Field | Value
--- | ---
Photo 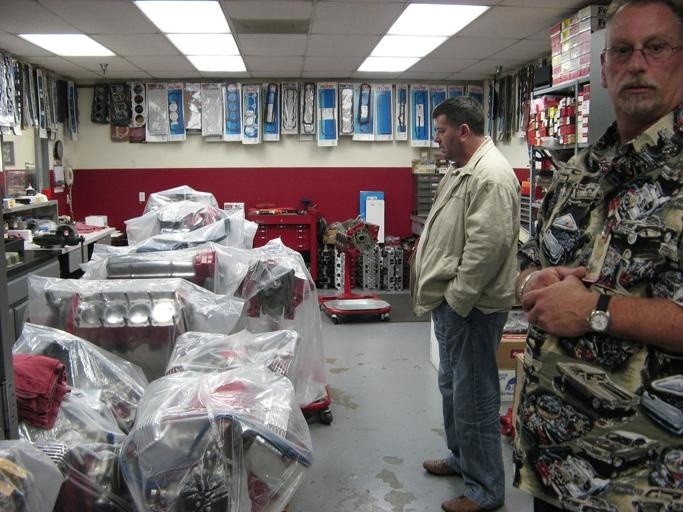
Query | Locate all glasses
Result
[601,40,682,63]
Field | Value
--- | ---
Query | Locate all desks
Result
[0,224,118,438]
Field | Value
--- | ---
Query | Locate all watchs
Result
[585,292,615,335]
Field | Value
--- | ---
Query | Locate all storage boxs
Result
[428,311,530,402]
[551,5,608,85]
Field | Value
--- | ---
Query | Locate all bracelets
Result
[516,268,539,300]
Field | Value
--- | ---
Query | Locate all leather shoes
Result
[442,495,484,511]
[424,457,461,476]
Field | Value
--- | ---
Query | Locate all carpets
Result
[325,292,432,324]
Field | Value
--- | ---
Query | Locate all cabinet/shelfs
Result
[519,193,532,232]
[244,209,318,289]
[525,75,593,252]
[415,173,445,216]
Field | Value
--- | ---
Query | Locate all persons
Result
[411,95,521,512]
[511,1,682,512]
